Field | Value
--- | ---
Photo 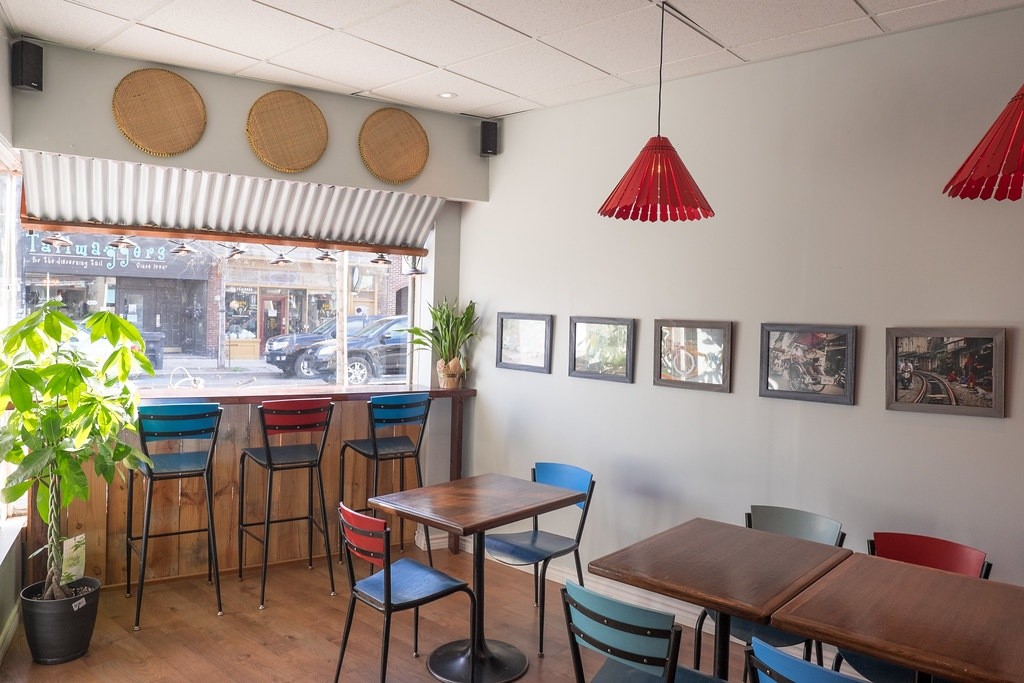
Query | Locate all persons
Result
[947,371,958,384]
[966,372,974,389]
[899,359,913,388]
[774,350,825,378]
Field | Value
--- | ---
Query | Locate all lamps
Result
[41,233,426,276]
[597,4,715,222]
[942,83,1024,201]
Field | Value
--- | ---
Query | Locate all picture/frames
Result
[495,313,1008,418]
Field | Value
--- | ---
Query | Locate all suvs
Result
[263,314,385,379]
[303,315,408,387]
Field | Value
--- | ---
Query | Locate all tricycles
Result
[780,343,827,392]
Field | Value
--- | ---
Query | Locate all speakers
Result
[12,40,43,91]
[478,121,497,156]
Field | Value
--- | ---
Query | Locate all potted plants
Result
[404,299,481,391]
[0,290,157,665]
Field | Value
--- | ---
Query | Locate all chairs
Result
[744,637,869,683]
[832,532,993,672]
[333,504,478,683]
[693,505,846,671]
[559,579,731,683]
[487,462,595,657]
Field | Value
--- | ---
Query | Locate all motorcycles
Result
[900,366,912,390]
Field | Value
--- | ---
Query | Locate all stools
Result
[237,398,336,610]
[340,393,435,577]
[125,403,223,631]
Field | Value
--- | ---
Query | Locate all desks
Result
[372,472,586,683]
[591,517,1024,683]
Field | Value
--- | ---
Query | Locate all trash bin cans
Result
[139,332,166,370]
[225,339,261,360]
